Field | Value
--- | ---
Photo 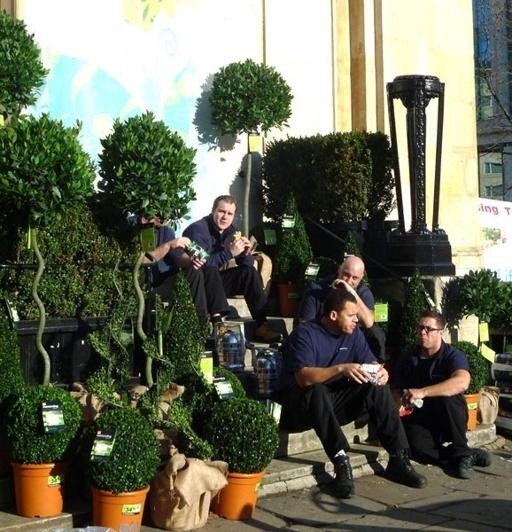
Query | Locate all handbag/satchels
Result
[149,452,228,531]
[478,386,499,424]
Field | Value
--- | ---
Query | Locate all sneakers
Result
[458,456,474,479]
[254,322,282,342]
[473,448,491,466]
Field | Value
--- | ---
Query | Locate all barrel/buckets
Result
[210,470,267,521]
[10,461,65,519]
[278,282,303,317]
[462,394,479,431]
[90,482,150,532]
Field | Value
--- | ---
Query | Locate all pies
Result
[232,230,241,241]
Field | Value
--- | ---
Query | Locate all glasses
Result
[416,324,442,333]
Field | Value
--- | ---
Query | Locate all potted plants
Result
[202,398,281,521]
[454,341,489,432]
[5,387,161,532]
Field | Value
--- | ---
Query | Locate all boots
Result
[384,452,426,488]
[333,456,355,499]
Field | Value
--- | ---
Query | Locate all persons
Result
[255,290,427,498]
[184,195,283,343]
[294,253,387,406]
[124,211,216,342]
[390,309,491,479]
